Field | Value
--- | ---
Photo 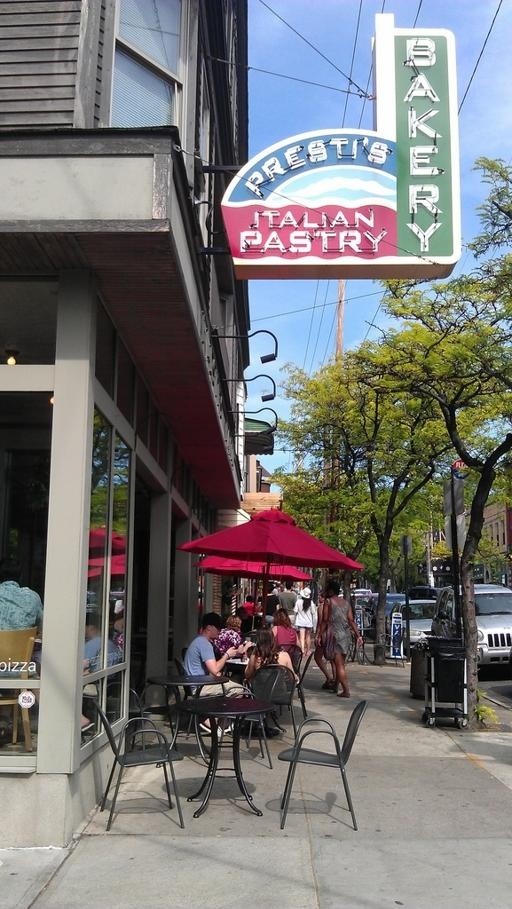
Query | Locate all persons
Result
[110,598,127,646]
[1,556,43,676]
[181,580,366,735]
[80,610,124,737]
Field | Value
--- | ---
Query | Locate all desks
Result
[175,695,276,818]
[224,655,250,685]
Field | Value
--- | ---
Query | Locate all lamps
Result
[5,349,19,365]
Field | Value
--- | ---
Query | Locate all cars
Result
[335,583,446,660]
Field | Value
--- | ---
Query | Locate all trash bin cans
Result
[426,637,466,703]
[410,642,431,700]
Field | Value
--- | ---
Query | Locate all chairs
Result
[235,663,298,749]
[277,700,369,831]
[278,652,316,716]
[180,647,188,661]
[89,698,185,831]
[106,654,148,751]
[269,644,303,716]
[0,628,36,753]
[242,665,281,769]
[173,657,234,738]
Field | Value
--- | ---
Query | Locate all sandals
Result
[322,678,336,689]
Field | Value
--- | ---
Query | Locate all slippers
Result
[199,722,221,737]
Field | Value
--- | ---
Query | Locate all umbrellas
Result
[194,554,310,601]
[85,554,126,580]
[89,526,130,551]
[177,507,364,626]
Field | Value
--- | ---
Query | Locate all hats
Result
[203,613,227,629]
[300,587,311,599]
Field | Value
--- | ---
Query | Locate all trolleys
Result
[421,629,470,730]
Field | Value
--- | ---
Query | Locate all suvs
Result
[427,584,512,671]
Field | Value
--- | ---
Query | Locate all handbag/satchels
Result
[322,597,336,660]
